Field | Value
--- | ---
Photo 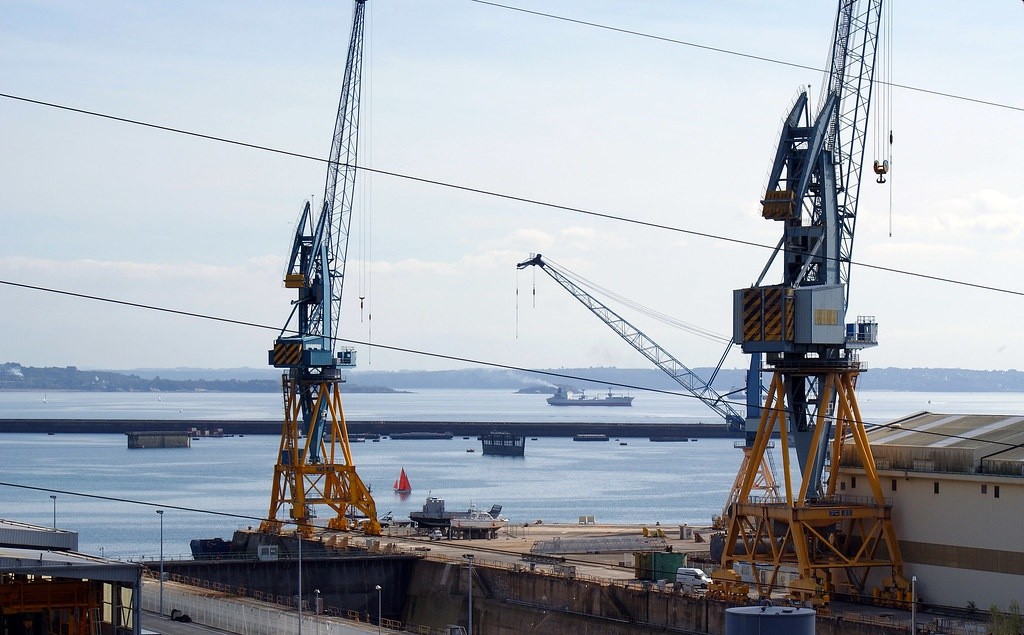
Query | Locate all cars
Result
[433,529,442,537]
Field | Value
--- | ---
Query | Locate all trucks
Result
[345,511,411,531]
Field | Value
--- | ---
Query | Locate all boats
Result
[286,427,388,444]
[545,386,636,407]
[725,383,770,401]
[649,426,690,442]
[573,432,610,441]
[390,430,454,440]
[409,490,510,532]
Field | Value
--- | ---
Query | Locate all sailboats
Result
[393,467,412,493]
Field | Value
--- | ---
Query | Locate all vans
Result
[675,567,713,588]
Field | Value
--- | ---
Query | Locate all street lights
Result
[157,509,164,616]
[375,585,382,635]
[462,553,474,635]
[293,530,303,635]
[50,495,57,528]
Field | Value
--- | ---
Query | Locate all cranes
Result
[259,0,385,536]
[514,253,798,537]
[702,0,921,618]
